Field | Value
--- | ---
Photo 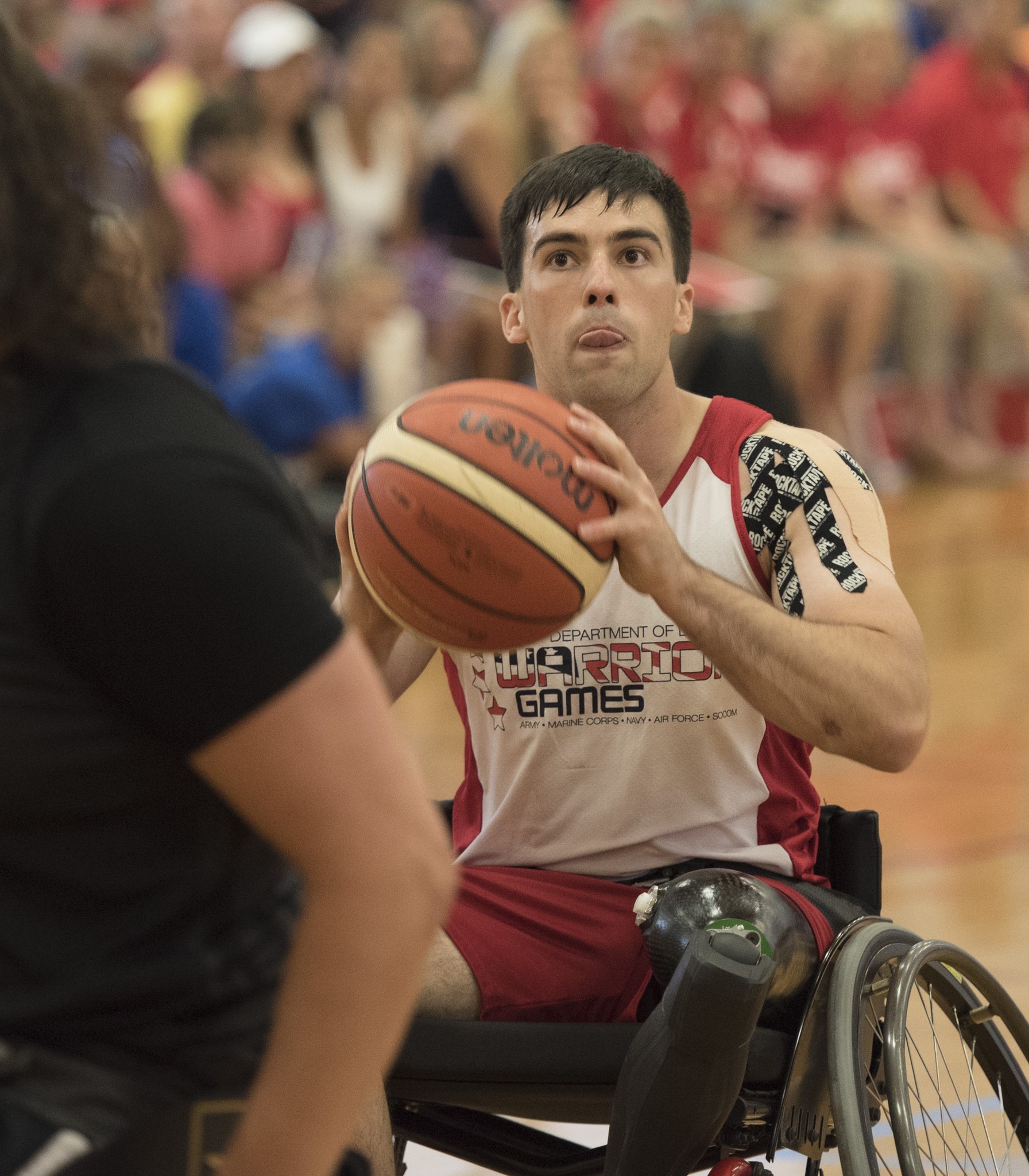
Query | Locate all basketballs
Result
[344,374,616,657]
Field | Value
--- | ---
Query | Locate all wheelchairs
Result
[386,798,1028,1176]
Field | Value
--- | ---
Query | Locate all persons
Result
[332,145,929,1176]
[14,0,1029,563]
[0,33,459,1176]
